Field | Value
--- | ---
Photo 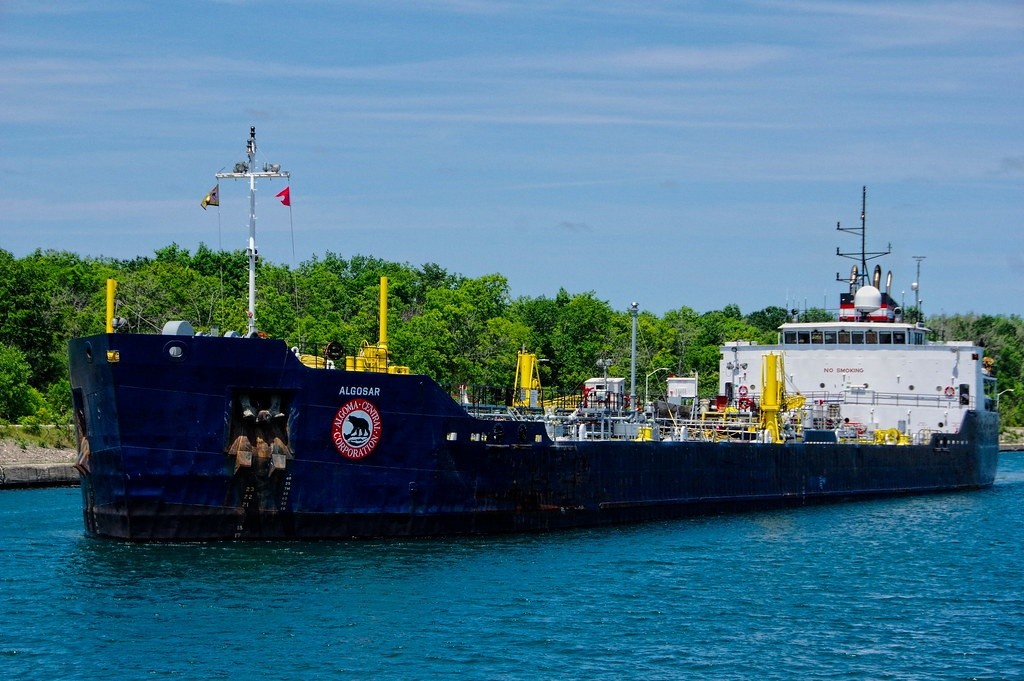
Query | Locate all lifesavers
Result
[738,396,750,409]
[739,385,746,395]
[945,386,955,397]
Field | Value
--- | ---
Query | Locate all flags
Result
[200,184,219,210]
[275,186,290,206]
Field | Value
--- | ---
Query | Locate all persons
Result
[982,357,995,377]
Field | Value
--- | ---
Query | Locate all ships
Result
[62,128,1002,544]
